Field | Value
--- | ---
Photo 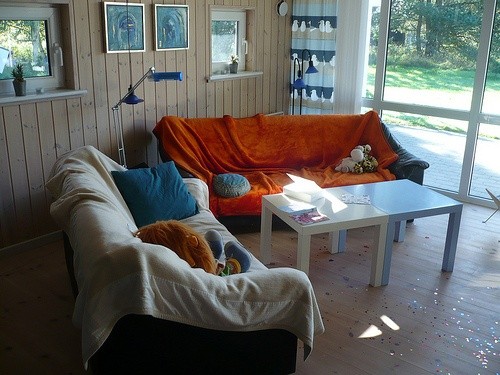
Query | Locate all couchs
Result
[152,110,432,234]
[41,145,326,375]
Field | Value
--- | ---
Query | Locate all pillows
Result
[110,160,201,230]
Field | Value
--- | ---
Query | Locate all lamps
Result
[290,48,319,116]
[111,66,183,168]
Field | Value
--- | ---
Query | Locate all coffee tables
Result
[259,179,465,289]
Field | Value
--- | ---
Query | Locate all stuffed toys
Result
[334,144,379,174]
[132,219,252,277]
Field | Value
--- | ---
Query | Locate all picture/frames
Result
[103,1,146,54]
[153,4,190,51]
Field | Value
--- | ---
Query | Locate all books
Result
[278,203,330,226]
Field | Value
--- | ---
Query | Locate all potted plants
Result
[228,55,240,75]
[10,63,27,97]
